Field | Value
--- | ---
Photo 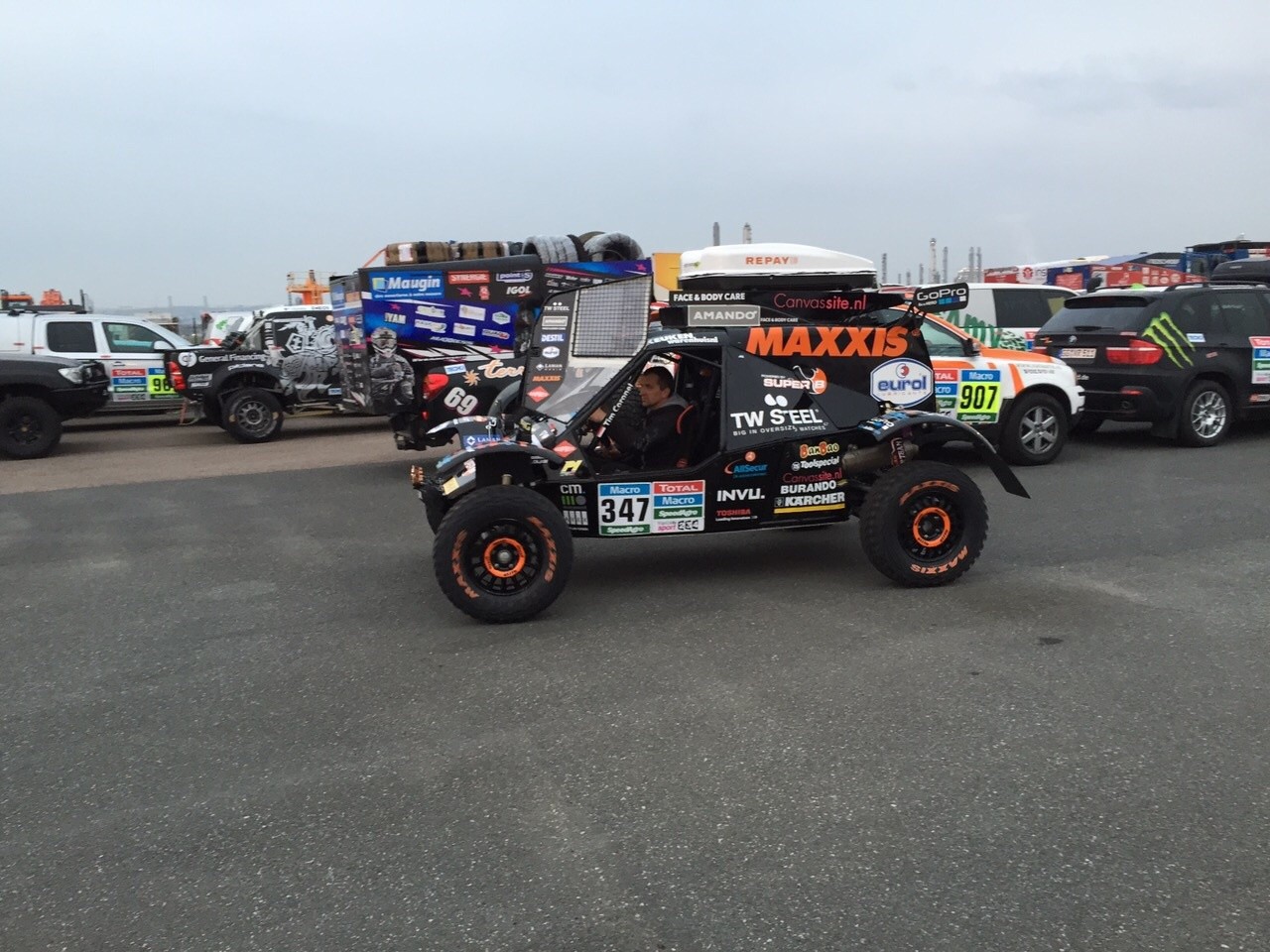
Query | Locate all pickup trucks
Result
[0,304,334,460]
[388,242,936,450]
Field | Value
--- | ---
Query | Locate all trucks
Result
[329,254,682,419]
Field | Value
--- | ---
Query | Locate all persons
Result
[575,365,693,476]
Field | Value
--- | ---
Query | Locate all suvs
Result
[876,258,1270,466]
[410,328,1031,625]
